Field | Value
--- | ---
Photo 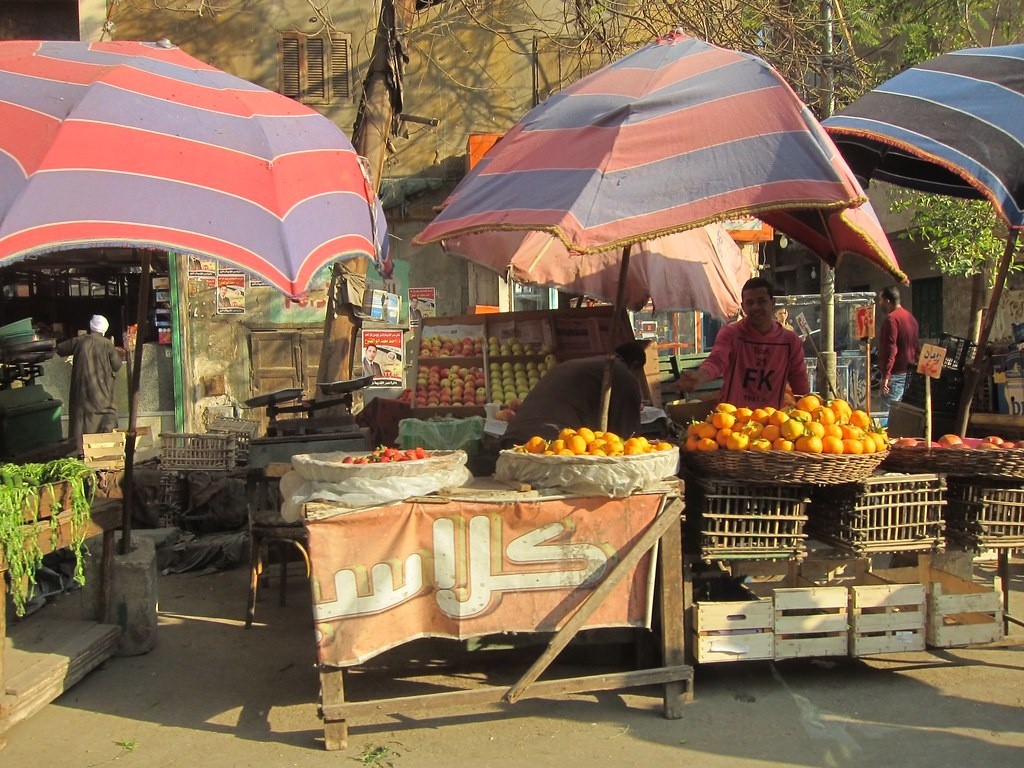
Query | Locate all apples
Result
[398,335,558,422]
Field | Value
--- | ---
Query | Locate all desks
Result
[304,477,693,750]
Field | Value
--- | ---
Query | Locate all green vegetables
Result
[0,456,99,615]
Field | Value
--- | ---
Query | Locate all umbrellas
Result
[0,37,394,553]
[410,28,911,439]
[817,42,1024,440]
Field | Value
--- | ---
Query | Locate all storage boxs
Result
[409,305,654,420]
[158,332,171,344]
[155,292,170,302]
[797,556,928,655]
[151,277,170,290]
[684,554,774,663]
[733,558,850,662]
[863,554,1006,648]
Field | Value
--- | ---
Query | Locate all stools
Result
[244,509,307,629]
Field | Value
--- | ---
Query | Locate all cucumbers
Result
[23,477,40,485]
[12,476,23,487]
[0,475,13,488]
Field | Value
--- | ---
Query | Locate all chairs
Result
[81,426,154,467]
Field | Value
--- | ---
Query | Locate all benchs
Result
[659,352,723,403]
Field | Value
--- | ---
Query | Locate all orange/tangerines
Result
[685,396,889,454]
[517,426,672,458]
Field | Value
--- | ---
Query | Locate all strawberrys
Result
[342,444,440,465]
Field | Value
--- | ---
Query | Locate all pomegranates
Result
[892,434,1024,450]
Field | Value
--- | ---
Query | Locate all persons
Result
[363,343,383,378]
[217,284,232,308]
[673,277,810,412]
[878,285,921,426]
[410,296,422,320]
[56,315,122,461]
[773,306,794,332]
[498,342,645,450]
